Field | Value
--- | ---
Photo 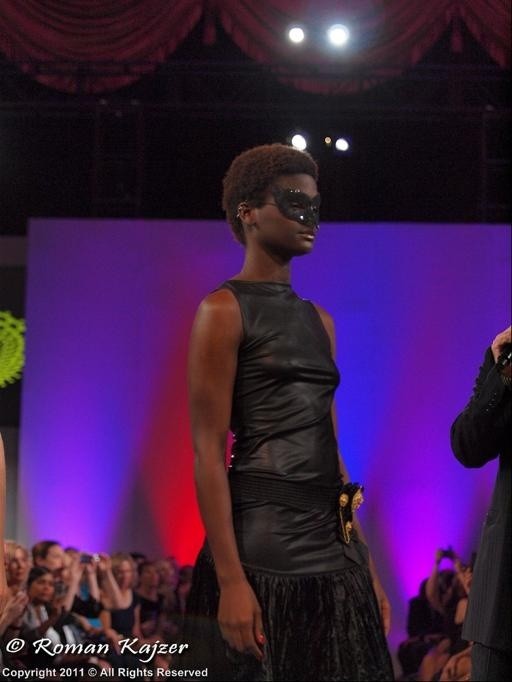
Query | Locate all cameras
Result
[79,555,93,565]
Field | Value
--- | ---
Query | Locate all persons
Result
[0,541,196,682]
[1,436,11,617]
[171,142,395,682]
[450,327,511,682]
[397,548,474,682]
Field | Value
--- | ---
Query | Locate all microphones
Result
[495,343,512,372]
